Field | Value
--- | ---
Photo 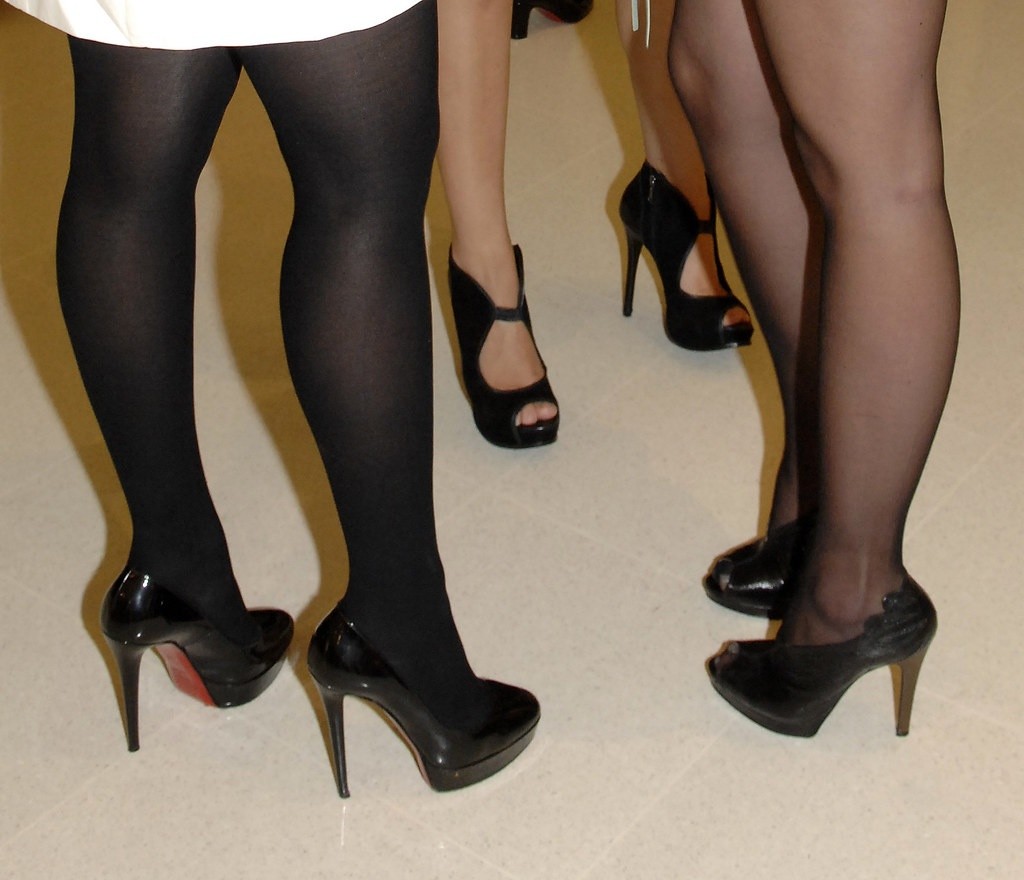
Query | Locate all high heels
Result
[703,514,815,619]
[307,603,541,799]
[449,240,560,448]
[509,0,592,40]
[705,569,937,739]
[618,160,754,351]
[101,565,294,751]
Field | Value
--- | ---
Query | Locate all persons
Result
[0,1,753,800]
[665,0,960,739]
